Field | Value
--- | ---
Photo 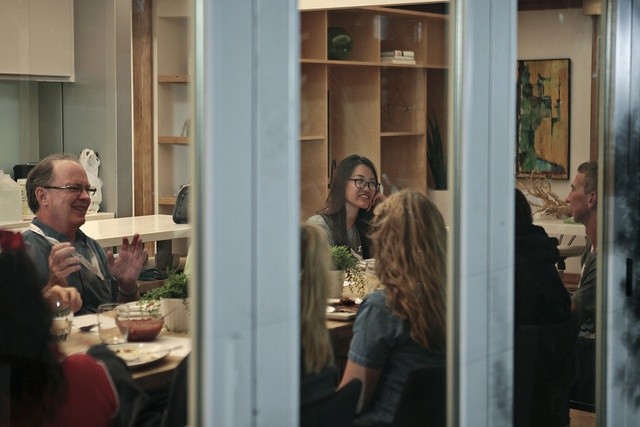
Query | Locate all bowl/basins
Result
[115,315,164,341]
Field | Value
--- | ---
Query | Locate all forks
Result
[75,321,103,332]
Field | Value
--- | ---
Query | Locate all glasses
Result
[45,184,97,196]
[348,177,381,191]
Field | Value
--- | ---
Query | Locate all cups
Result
[49,296,74,342]
[97,303,130,344]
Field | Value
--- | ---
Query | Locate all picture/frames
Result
[516,58,572,180]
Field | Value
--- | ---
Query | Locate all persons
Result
[44,285,84,316]
[305,155,389,271]
[337,187,447,416]
[513,189,570,425]
[88,221,334,427]
[1,229,119,427]
[16,154,148,315]
[567,161,597,328]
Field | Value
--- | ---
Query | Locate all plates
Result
[326,296,363,321]
[106,342,169,367]
[114,301,159,312]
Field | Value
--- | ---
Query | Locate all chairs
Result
[300,377,362,426]
[391,366,446,427]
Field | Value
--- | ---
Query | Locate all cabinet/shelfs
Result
[299,7,452,223]
[1,0,76,85]
[133,0,188,272]
